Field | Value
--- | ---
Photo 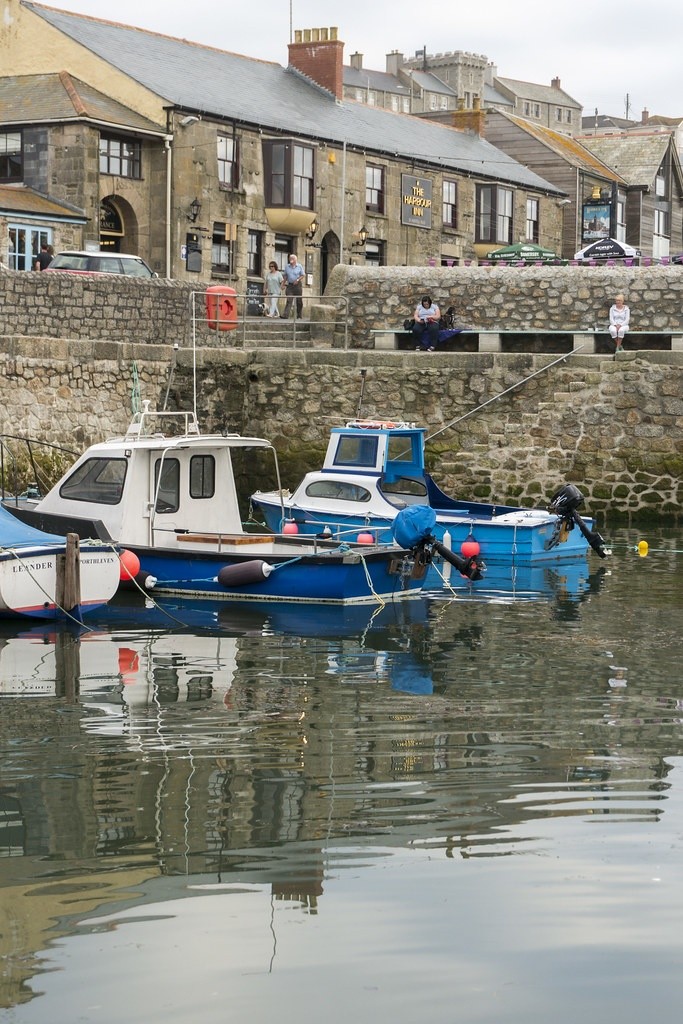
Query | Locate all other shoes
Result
[267,315,272,318]
[280,316,288,319]
[415,346,421,351]
[427,348,433,352]
[297,316,302,319]
[619,346,624,351]
[615,348,619,353]
[274,316,280,318]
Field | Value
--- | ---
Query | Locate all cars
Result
[42,250,161,279]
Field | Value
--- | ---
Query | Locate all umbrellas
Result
[574,236,643,260]
[490,241,563,260]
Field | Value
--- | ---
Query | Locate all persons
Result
[263,254,306,319]
[414,296,441,351]
[35,243,54,271]
[609,293,630,354]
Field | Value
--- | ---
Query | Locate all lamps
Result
[558,199,571,207]
[178,115,199,128]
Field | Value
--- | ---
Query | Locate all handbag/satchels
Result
[404,319,415,330]
[439,307,456,332]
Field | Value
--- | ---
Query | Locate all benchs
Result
[370,328,683,354]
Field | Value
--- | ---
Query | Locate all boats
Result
[0,499,144,624]
[247,416,612,569]
[0,292,488,610]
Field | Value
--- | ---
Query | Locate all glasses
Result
[269,266,274,267]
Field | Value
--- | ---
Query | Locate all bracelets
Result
[297,279,300,282]
